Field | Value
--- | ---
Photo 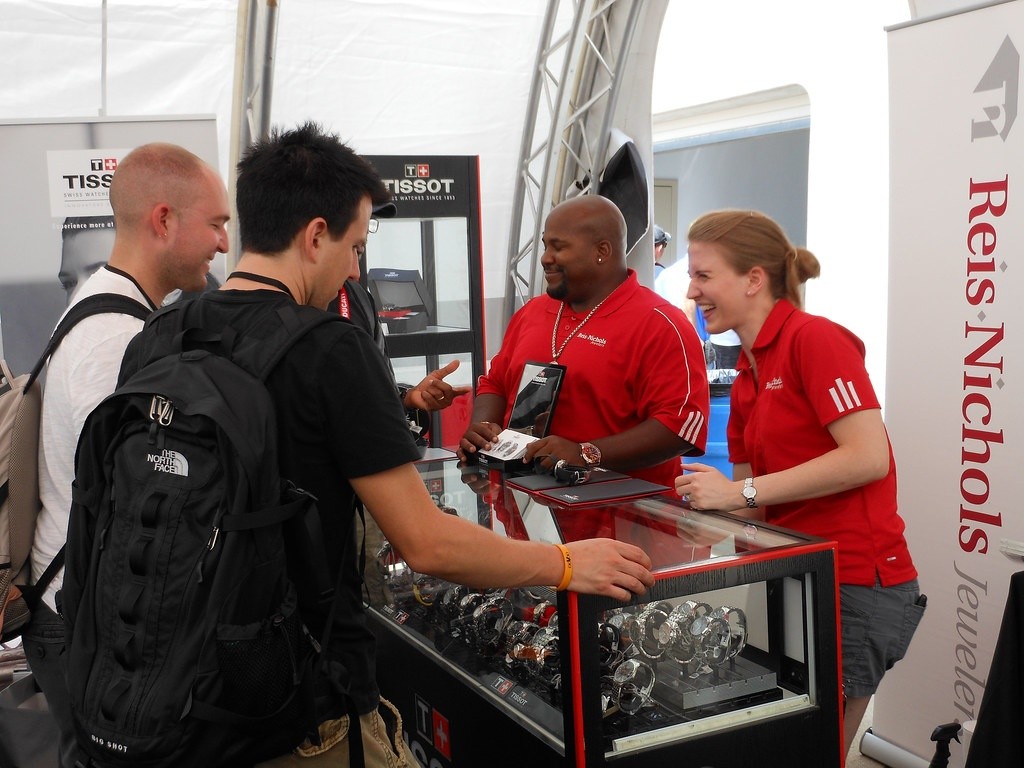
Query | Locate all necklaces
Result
[549,302,602,365]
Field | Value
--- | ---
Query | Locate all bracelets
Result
[480,421,489,423]
[550,544,572,591]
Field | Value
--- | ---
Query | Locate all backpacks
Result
[55,298,359,767]
[0,294,155,645]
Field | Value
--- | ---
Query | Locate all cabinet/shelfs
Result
[356,154,487,453]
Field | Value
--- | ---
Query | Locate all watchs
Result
[742,524,757,550]
[580,442,601,467]
[378,539,748,714]
[741,477,758,508]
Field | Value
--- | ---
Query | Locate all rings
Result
[438,396,444,400]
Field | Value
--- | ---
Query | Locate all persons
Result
[456,195,710,501]
[675,211,926,763]
[654,224,675,299]
[328,202,472,412]
[685,299,711,342]
[20,141,231,768]
[456,460,712,568]
[57,216,223,307]
[144,122,656,768]
